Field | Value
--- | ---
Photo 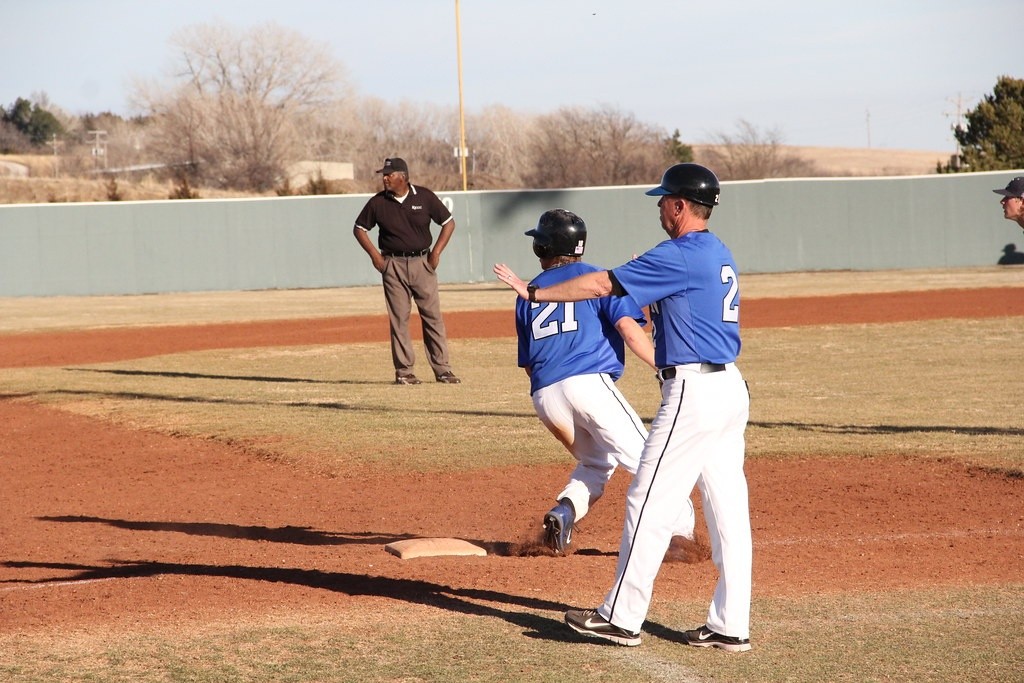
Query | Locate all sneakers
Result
[436,371,460,384]
[544,500,580,554]
[565,609,641,647]
[395,374,421,385]
[682,625,751,653]
[665,532,712,562]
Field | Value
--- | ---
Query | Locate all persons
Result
[354,158,460,385]
[992,177,1024,231]
[514,208,694,552]
[493,164,754,651]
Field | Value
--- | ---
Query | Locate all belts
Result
[657,363,725,381]
[382,250,429,257]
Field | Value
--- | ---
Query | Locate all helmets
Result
[645,163,719,206]
[524,209,587,259]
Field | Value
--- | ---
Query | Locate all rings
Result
[508,275,511,280]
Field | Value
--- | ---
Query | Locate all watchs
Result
[527,285,540,303]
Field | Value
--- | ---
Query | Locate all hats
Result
[376,158,408,174]
[993,177,1024,198]
